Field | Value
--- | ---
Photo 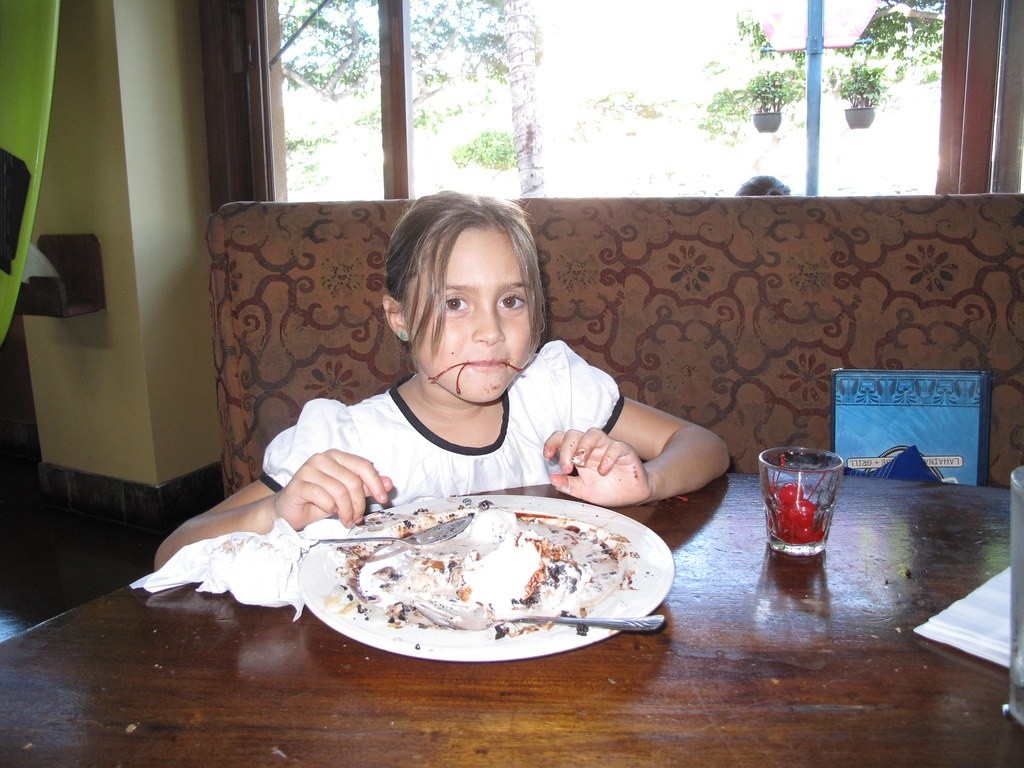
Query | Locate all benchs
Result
[207,193,1024,507]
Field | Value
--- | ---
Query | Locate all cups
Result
[759,447,845,555]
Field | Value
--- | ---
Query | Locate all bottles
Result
[1008,466,1024,724]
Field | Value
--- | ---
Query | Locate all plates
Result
[298,496,675,663]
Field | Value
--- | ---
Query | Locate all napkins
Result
[128,519,317,623]
[911,569,1011,666]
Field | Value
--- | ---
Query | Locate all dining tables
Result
[0,502,1024,768]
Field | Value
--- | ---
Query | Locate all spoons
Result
[309,515,472,546]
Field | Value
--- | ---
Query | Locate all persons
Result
[153,189,731,573]
[735,175,790,196]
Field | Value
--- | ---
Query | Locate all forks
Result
[413,599,665,632]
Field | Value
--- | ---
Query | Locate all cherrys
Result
[767,452,828,538]
